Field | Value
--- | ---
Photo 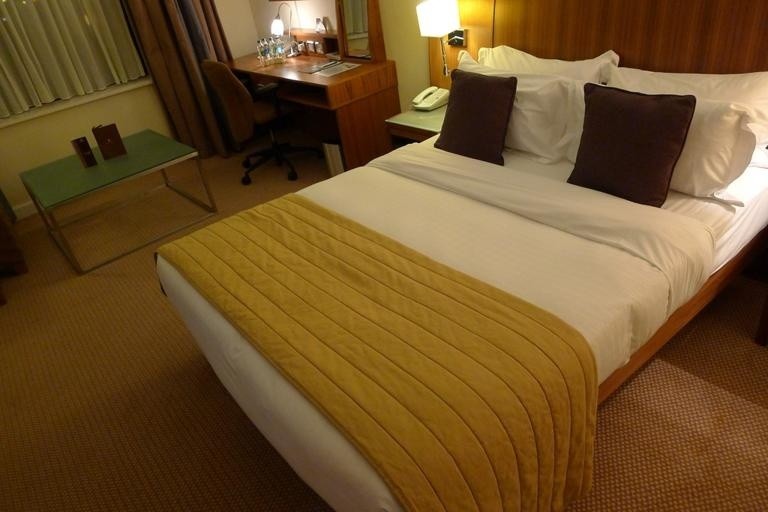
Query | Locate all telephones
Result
[411,86,450,111]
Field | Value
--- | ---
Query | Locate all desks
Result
[221,48,401,171]
[19,129,219,274]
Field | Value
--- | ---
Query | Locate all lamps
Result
[270,2,301,58]
[416,0,467,76]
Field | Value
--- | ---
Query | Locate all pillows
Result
[457,50,579,165]
[567,82,696,208]
[571,82,756,207]
[478,44,619,98]
[606,60,768,170]
[434,69,517,166]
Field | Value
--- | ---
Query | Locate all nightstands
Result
[384,103,449,155]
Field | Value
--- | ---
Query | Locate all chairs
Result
[202,60,325,185]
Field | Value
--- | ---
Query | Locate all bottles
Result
[256,36,286,66]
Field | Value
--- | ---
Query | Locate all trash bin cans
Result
[321,136,346,177]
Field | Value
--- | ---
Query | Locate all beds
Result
[153,132,768,512]
